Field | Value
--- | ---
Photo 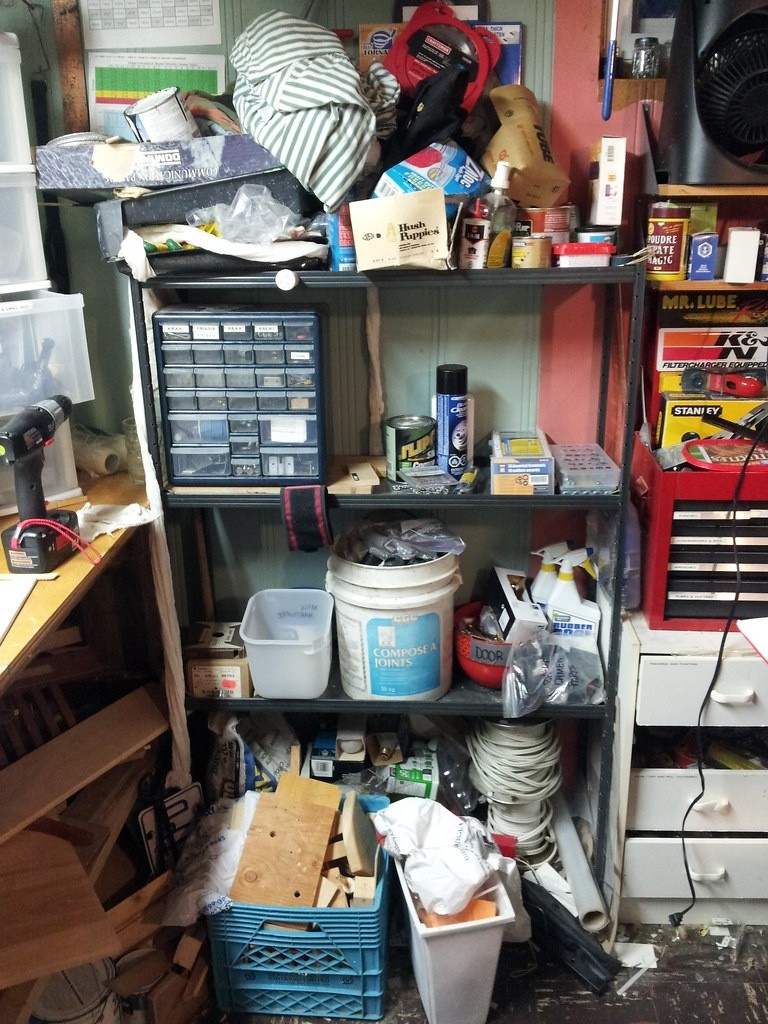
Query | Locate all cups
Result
[121,418,145,485]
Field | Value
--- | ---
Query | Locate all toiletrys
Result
[477,161,517,232]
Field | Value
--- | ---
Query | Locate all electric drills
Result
[0,396,103,574]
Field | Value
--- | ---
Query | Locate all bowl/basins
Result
[455,602,511,689]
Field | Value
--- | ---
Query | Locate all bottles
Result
[632,36,671,79]
[529,541,601,645]
[456,161,515,269]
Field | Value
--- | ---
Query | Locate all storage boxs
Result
[647,301,767,459]
[308,713,441,802]
[120,133,303,229]
[239,588,335,700]
[184,618,254,700]
[586,133,628,225]
[370,133,494,225]
[201,793,392,1019]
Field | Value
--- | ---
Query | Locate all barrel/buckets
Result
[123,86,201,140]
[30,948,171,1023]
[326,547,463,701]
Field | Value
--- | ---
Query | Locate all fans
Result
[653,0,767,189]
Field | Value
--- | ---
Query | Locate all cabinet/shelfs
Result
[132,218,649,896]
[622,180,768,930]
[0,32,99,515]
[0,468,145,1024]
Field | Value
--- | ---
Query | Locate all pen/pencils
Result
[601,0,621,121]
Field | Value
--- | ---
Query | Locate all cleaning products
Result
[530,539,598,617]
